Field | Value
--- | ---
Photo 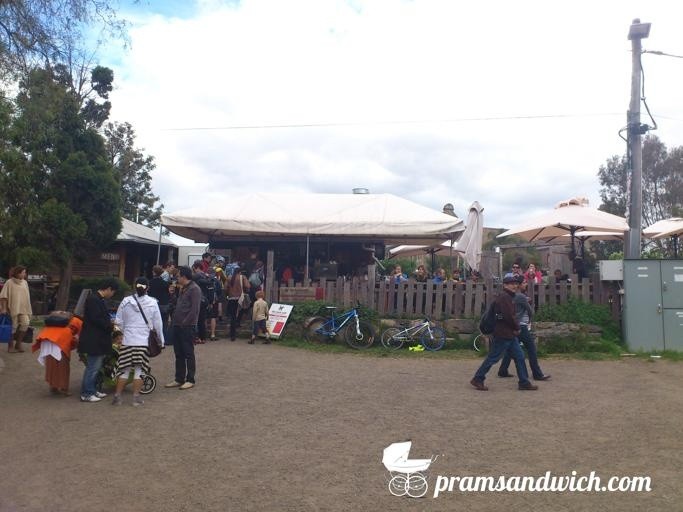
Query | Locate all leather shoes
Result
[533,374,550,381]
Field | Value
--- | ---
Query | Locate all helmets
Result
[502,272,517,283]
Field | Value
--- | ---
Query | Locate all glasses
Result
[512,266,519,269]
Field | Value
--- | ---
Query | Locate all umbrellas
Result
[452,201,485,276]
[496,197,631,269]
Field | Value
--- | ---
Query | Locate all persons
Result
[111,276,164,408]
[147,251,315,345]
[375,261,572,316]
[32,310,83,396]
[0,265,33,353]
[111,331,124,350]
[77,276,119,402]
[497,276,551,381]
[469,273,539,391]
[164,266,202,390]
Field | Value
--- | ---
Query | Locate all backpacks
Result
[44,310,72,328]
[478,300,498,336]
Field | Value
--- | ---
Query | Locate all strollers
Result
[75,339,157,394]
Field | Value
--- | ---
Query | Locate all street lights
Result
[441,203,459,275]
[624,18,652,261]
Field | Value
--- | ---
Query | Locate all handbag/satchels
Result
[15,323,34,343]
[147,328,162,358]
[236,292,251,310]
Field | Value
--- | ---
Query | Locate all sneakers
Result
[163,380,182,388]
[469,377,488,392]
[111,394,123,407]
[80,391,107,402]
[209,336,216,341]
[262,339,271,344]
[131,394,144,407]
[517,383,537,391]
[178,382,195,390]
[499,373,513,378]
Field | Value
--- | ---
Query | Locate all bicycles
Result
[381,315,446,351]
[304,299,375,350]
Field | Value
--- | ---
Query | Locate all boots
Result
[7,333,16,354]
[15,330,26,353]
[247,338,255,344]
